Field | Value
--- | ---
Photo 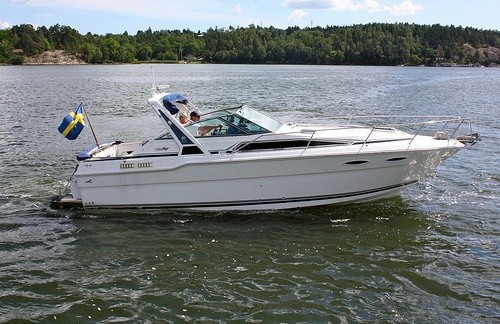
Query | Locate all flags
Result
[58,104,86,139]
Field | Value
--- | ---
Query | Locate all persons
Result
[179,114,188,124]
[190,110,223,136]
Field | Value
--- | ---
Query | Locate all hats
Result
[190,111,200,117]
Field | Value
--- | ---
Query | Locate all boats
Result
[50,83,481,214]
